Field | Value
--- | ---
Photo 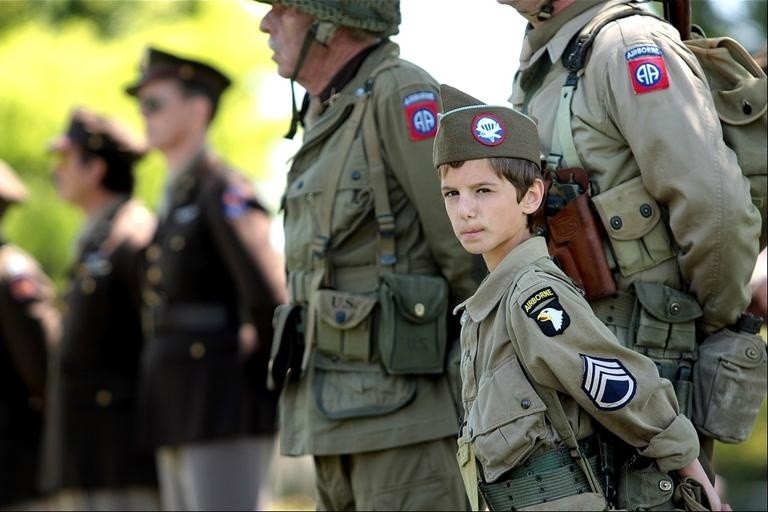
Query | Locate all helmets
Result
[253,0,402,35]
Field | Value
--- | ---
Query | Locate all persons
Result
[497,2,767,467]
[430,83,731,510]
[256,0,475,511]
[56,110,159,512]
[1,161,62,511]
[744,244,768,334]
[138,46,290,512]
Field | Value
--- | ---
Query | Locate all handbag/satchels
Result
[596,425,714,512]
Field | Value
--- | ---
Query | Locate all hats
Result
[432,84,542,172]
[126,47,231,97]
[52,107,146,162]
[0,159,26,202]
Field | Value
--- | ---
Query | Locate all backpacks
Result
[559,4,768,253]
